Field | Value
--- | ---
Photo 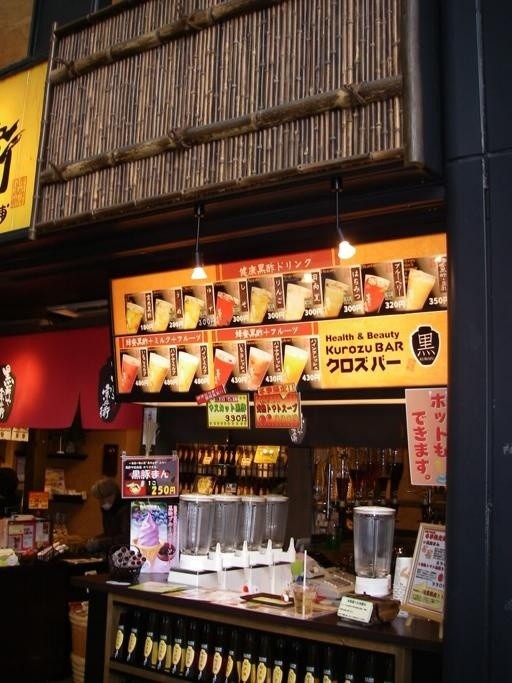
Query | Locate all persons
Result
[86,478,130,575]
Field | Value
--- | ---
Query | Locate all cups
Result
[127,268,437,333]
[57,431,65,454]
[291,581,317,617]
[121,345,308,393]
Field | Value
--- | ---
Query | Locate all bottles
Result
[171,444,287,495]
[111,605,395,682]
[337,447,404,499]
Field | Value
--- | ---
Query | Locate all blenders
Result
[167,493,297,593]
[351,504,396,596]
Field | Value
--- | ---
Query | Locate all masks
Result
[102,503,112,508]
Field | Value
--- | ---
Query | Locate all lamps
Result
[192,202,206,279]
[332,175,355,259]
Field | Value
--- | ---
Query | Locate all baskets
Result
[108,545,142,583]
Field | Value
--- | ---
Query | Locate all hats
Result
[91,478,118,497]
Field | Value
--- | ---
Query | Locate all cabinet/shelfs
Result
[70,573,446,683]
